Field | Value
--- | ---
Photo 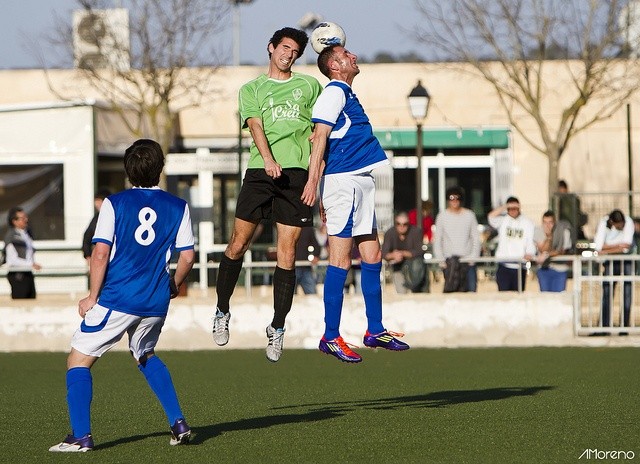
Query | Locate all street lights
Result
[405,76,434,294]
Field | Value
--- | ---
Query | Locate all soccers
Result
[310,21,347,54]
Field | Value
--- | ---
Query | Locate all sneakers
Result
[265,324,285,361]
[363,329,410,350]
[169,419,192,446]
[319,335,362,363]
[48,434,94,453]
[212,307,231,345]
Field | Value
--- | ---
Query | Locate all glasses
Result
[449,194,460,201]
[395,223,408,226]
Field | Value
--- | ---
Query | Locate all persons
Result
[49,139,195,452]
[212,27,326,362]
[487,197,536,292]
[82,189,112,290]
[2,206,41,299]
[300,42,410,364]
[558,180,587,255]
[382,212,429,293]
[250,218,273,286]
[292,225,327,294]
[432,186,482,293]
[408,200,434,245]
[533,210,572,293]
[588,210,637,337]
